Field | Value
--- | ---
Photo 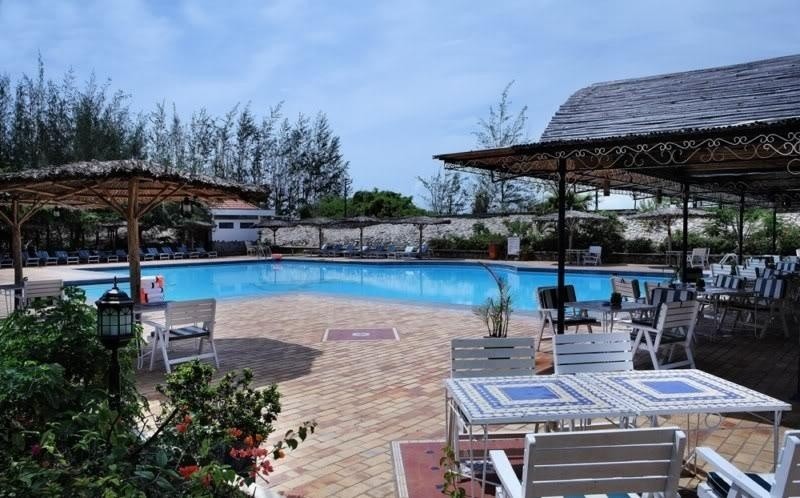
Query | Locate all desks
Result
[442,374,634,497]
[575,369,792,498]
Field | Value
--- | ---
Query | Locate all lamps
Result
[181,196,196,219]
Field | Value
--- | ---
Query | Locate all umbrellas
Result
[0,159,276,330]
[249,220,295,248]
[330,216,386,251]
[531,205,608,250]
[1,171,62,311]
[170,220,217,249]
[293,216,336,248]
[626,206,718,265]
[382,216,451,254]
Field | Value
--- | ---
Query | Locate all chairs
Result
[695,429,799,497]
[447,338,553,476]
[245,240,272,257]
[13,279,64,322]
[551,331,637,433]
[304,238,435,260]
[1,244,218,268]
[489,426,686,498]
[536,244,800,370]
[149,299,221,376]
